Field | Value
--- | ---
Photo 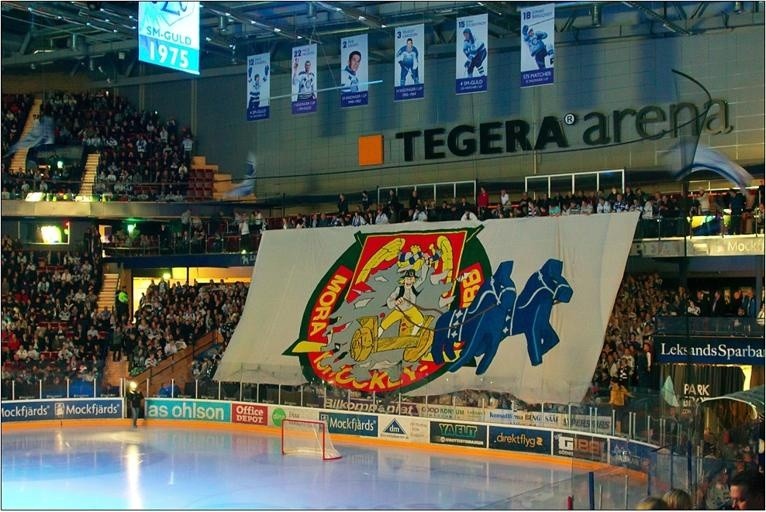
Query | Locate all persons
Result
[341,50,362,92]
[520,24,555,70]
[292,57,318,101]
[127,386,145,430]
[247,64,270,109]
[461,28,488,80]
[1,87,237,420]
[238,179,766,510]
[395,37,420,85]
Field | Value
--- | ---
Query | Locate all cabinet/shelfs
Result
[188,155,256,202]
